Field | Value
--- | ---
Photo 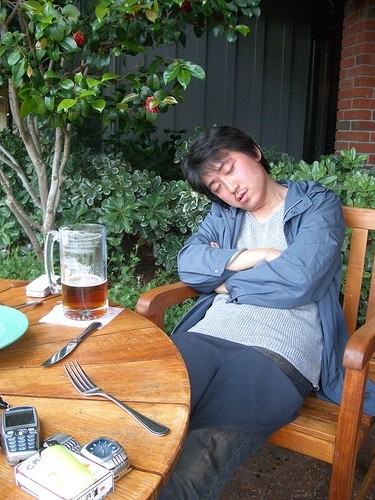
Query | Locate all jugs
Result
[43,223,109,319]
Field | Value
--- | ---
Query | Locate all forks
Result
[63,360,169,437]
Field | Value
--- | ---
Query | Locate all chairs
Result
[136,205,375,499]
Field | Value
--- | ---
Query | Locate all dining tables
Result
[0,278,191,500]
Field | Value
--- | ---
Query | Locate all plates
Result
[0,305,29,349]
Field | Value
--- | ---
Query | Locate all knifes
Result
[40,322,102,366]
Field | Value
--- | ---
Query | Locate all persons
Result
[156,125,375,500]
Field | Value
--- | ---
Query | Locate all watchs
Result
[41,433,133,483]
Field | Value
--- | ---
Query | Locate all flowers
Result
[72,1,193,113]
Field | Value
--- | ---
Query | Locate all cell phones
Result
[2,406,40,465]
[25,275,61,297]
